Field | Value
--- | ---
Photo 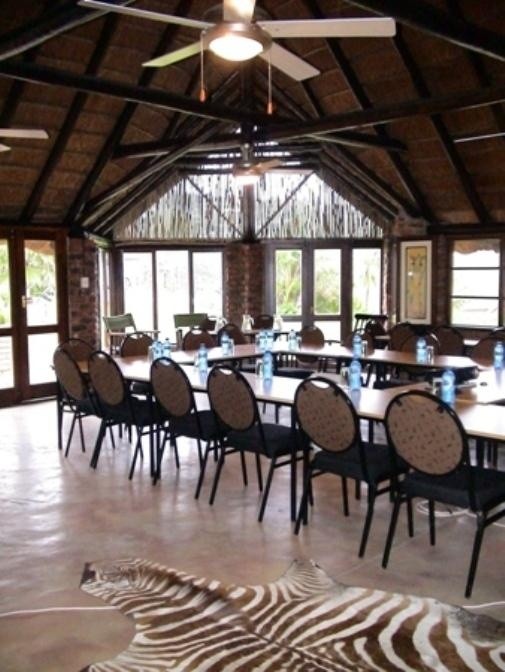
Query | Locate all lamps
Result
[234,175,261,185]
[200,18,274,62]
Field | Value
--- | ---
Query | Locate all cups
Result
[241,314,254,333]
[194,352,199,372]
[433,377,442,398]
[255,334,260,352]
[272,314,283,331]
[215,314,227,333]
[229,339,235,355]
[255,359,262,379]
[147,346,154,364]
[296,337,302,349]
[362,340,368,357]
[427,346,434,362]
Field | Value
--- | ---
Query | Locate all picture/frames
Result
[395,236,437,325]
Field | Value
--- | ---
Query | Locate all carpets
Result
[78,557,505,671]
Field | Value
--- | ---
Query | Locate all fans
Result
[209,123,285,187]
[77,0,398,83]
[0,129,50,153]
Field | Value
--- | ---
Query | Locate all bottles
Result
[163,338,171,358]
[440,367,457,404]
[263,379,273,394]
[352,333,362,354]
[266,329,274,348]
[351,390,361,411]
[494,341,505,368]
[259,328,267,348]
[262,350,273,379]
[415,336,427,361]
[220,332,229,355]
[288,329,297,349]
[348,355,362,390]
[198,342,207,372]
[199,372,209,384]
[155,341,163,360]
